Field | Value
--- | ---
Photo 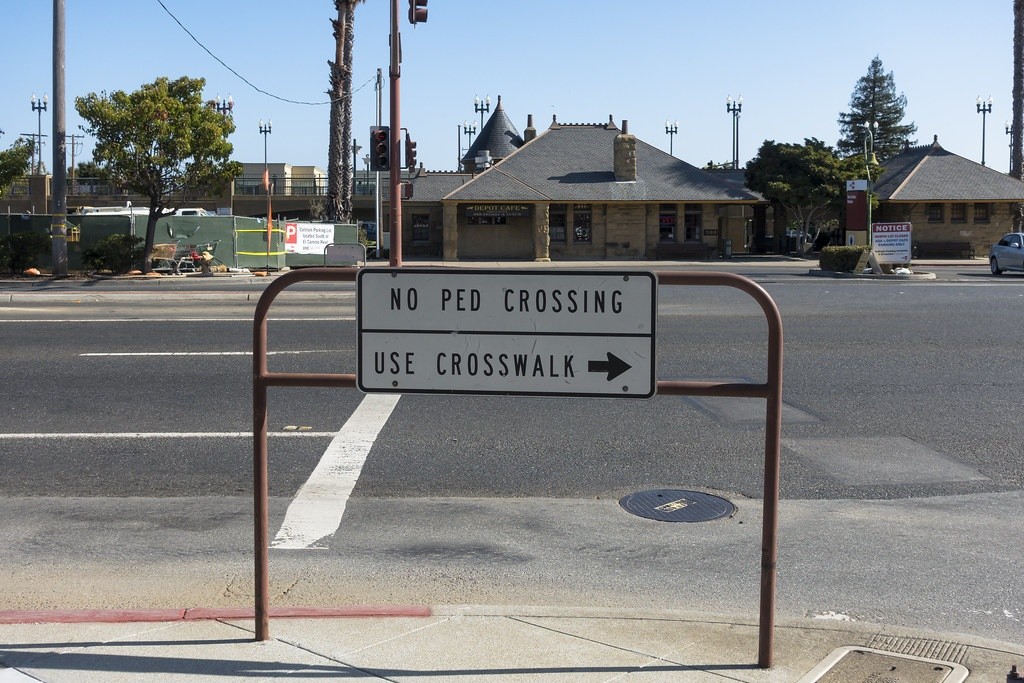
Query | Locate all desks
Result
[656,241,708,261]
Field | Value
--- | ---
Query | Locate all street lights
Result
[976,94,992,167]
[215,94,233,166]
[1006,120,1017,174]
[474,93,489,131]
[30,91,48,173]
[464,120,476,151]
[735,111,741,169]
[861,120,879,248]
[726,93,742,169]
[257,118,272,218]
[665,120,679,157]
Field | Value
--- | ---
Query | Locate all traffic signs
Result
[354,268,659,400]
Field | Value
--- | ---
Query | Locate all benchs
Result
[914,240,975,259]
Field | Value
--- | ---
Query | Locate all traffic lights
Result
[370,126,389,171]
[408,0,428,27]
[405,134,417,172]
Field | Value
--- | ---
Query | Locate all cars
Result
[989,232,1024,276]
